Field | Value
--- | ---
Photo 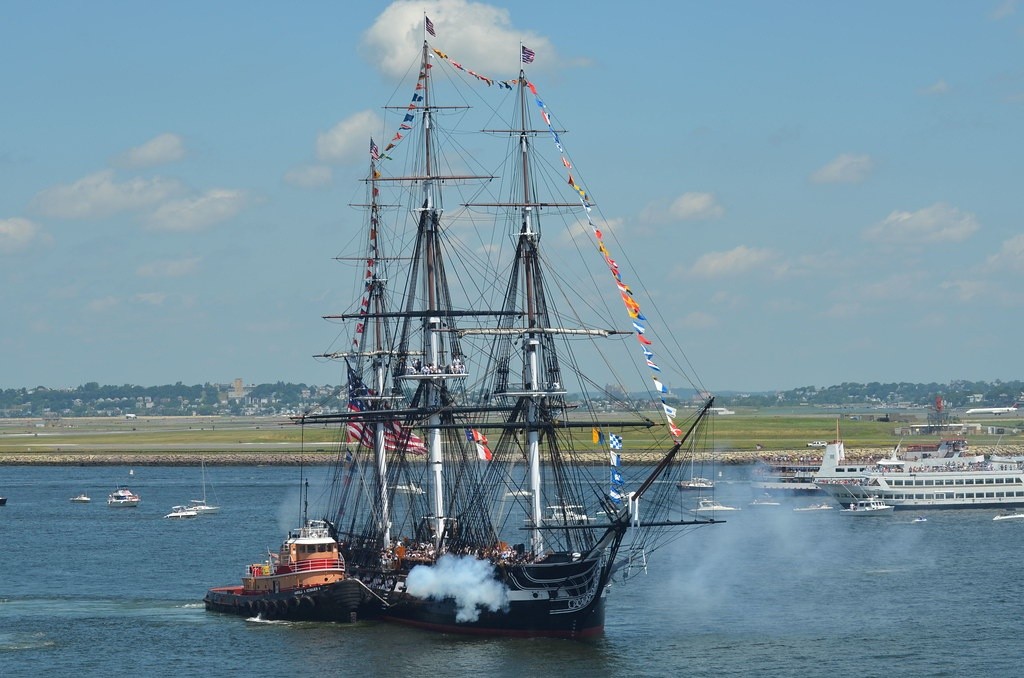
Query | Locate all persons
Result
[850,502,858,510]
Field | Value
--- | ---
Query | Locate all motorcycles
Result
[914,517,927,523]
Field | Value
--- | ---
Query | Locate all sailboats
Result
[289,10,724,638]
[189,455,220,514]
[675,427,716,490]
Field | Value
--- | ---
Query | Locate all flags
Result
[345,358,429,455]
[344,448,358,487]
[464,429,493,461]
[522,46,535,63]
[352,42,682,437]
[756,444,763,451]
[592,427,625,504]
[370,139,379,159]
[425,16,436,37]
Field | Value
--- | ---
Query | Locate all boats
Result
[69,495,91,503]
[596,509,622,516]
[792,504,834,513]
[387,481,426,495]
[876,458,905,467]
[0,497,8,505]
[523,497,597,526]
[690,500,742,516]
[746,459,876,495]
[506,488,532,497]
[993,511,1024,521]
[839,498,895,516]
[810,417,1024,510]
[106,488,141,508]
[746,495,781,509]
[163,506,198,518]
[203,525,364,624]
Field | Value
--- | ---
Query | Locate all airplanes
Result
[966,402,1019,415]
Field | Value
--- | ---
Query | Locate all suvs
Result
[806,441,827,447]
